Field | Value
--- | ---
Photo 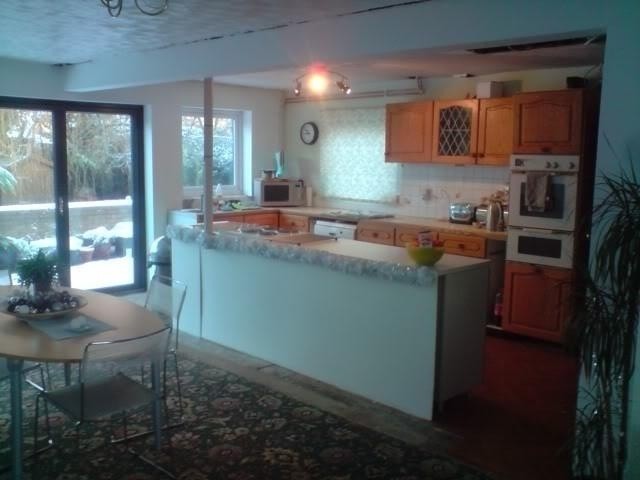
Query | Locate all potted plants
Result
[15,248,68,292]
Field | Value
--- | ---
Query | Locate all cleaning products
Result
[305,185,314,208]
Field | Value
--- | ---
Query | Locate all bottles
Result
[216,183,224,201]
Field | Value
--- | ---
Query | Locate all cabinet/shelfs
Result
[279,214,316,232]
[384,99,433,164]
[502,260,587,349]
[215,214,279,229]
[432,96,513,165]
[513,89,598,156]
[356,220,505,260]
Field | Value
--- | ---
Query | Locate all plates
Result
[0,293,89,322]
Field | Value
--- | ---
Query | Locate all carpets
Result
[0,351,509,480]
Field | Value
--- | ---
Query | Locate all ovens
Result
[309,219,357,240]
[507,152,581,269]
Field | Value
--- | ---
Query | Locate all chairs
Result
[0,273,187,465]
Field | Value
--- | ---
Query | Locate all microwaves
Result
[253,177,304,208]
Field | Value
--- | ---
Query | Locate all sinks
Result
[186,207,202,214]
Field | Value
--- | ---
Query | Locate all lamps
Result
[293,71,352,96]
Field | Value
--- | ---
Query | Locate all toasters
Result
[450,202,475,225]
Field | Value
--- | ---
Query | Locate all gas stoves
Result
[318,208,395,222]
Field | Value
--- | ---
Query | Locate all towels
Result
[524,171,552,214]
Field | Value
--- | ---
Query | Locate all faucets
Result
[198,191,205,211]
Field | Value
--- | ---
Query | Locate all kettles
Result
[486,199,505,233]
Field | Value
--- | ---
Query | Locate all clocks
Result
[300,121,318,145]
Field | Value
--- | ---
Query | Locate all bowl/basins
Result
[405,242,446,267]
